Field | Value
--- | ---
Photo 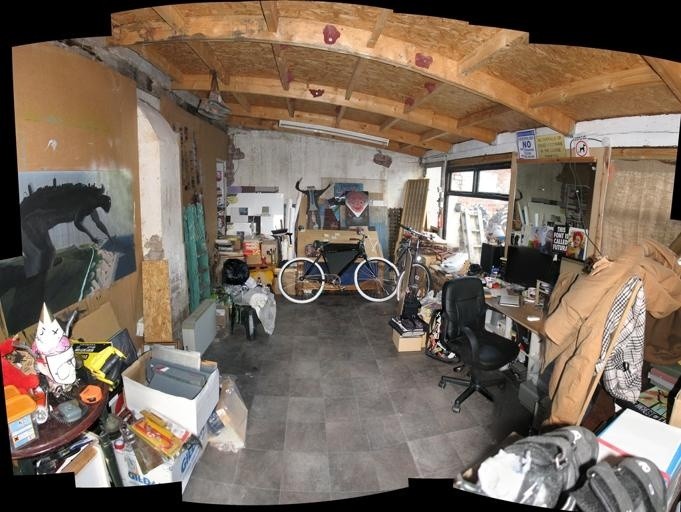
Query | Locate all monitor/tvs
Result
[505,245,565,290]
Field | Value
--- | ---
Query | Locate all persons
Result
[567,230,585,261]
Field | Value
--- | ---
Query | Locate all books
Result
[483,291,493,300]
[439,269,453,278]
[498,293,521,308]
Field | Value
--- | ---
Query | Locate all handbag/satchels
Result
[426,309,459,364]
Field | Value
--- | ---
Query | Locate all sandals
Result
[474,425,667,512]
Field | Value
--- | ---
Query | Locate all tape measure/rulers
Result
[79,384,103,404]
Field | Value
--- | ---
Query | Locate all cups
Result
[526,287,536,298]
[39,343,77,384]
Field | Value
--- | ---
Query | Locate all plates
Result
[272,229,287,233]
[524,297,536,303]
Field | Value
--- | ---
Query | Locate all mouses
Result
[527,316,541,322]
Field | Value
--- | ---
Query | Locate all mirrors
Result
[510,155,602,262]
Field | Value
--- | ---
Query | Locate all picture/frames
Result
[278,119,390,146]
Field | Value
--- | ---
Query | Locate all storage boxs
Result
[112,348,249,497]
[392,330,422,352]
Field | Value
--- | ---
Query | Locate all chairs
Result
[438,277,520,413]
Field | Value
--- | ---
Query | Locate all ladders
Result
[460,207,486,263]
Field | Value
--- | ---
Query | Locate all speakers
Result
[481,242,505,273]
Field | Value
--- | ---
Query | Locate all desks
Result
[10,382,110,461]
[485,296,548,415]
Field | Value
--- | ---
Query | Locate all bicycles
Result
[396,220,433,301]
[277,233,399,304]
